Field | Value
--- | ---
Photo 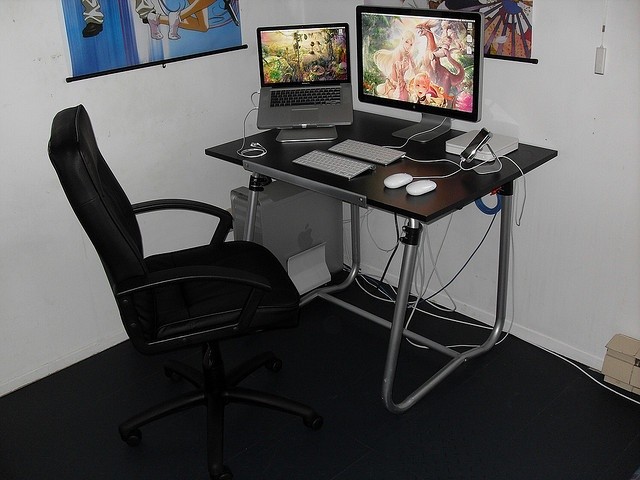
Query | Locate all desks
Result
[205,110,558,415]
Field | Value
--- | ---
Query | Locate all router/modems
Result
[447,126,517,164]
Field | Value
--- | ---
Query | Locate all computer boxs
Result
[230,181,343,270]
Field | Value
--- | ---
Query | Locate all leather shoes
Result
[143,12,156,23]
[83,23,103,37]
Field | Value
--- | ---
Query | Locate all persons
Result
[408,73,451,107]
[374,32,417,102]
[81,0,156,38]
[147,0,218,40]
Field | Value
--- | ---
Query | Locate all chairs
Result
[47,103,325,479]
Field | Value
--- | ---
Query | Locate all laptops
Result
[255,25,354,128]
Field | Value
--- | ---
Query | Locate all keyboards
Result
[331,134,405,164]
[291,146,371,183]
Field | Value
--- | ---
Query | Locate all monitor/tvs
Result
[355,4,483,145]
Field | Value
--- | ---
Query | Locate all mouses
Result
[406,172,436,199]
[382,171,413,189]
